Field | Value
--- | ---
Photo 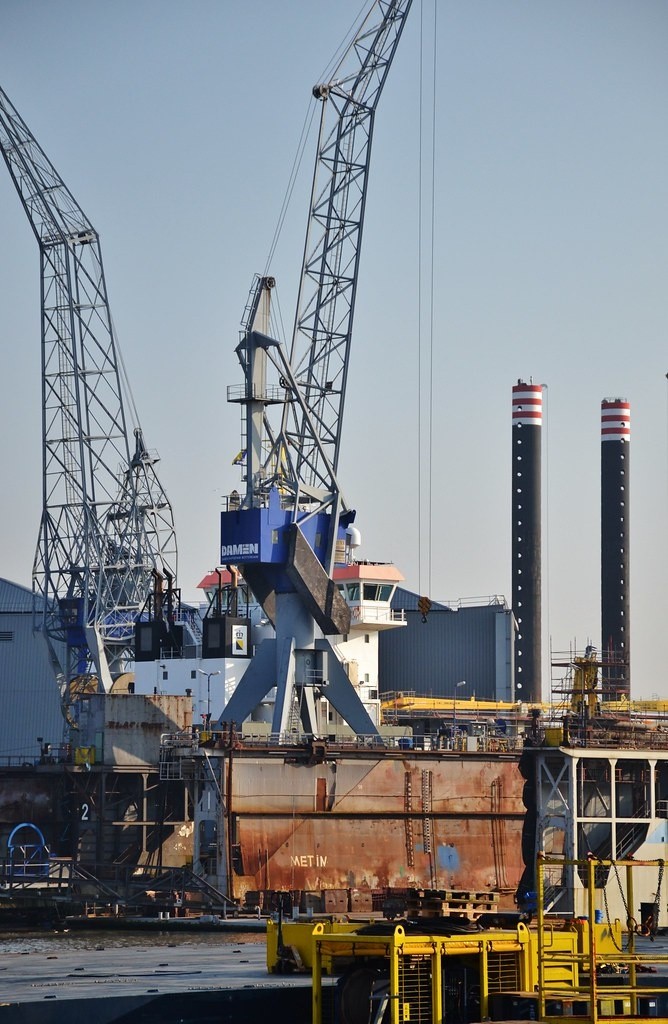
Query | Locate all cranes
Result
[0,88,179,765]
[187,2,409,739]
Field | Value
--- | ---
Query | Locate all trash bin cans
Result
[638,902,659,934]
[640,996,659,1016]
